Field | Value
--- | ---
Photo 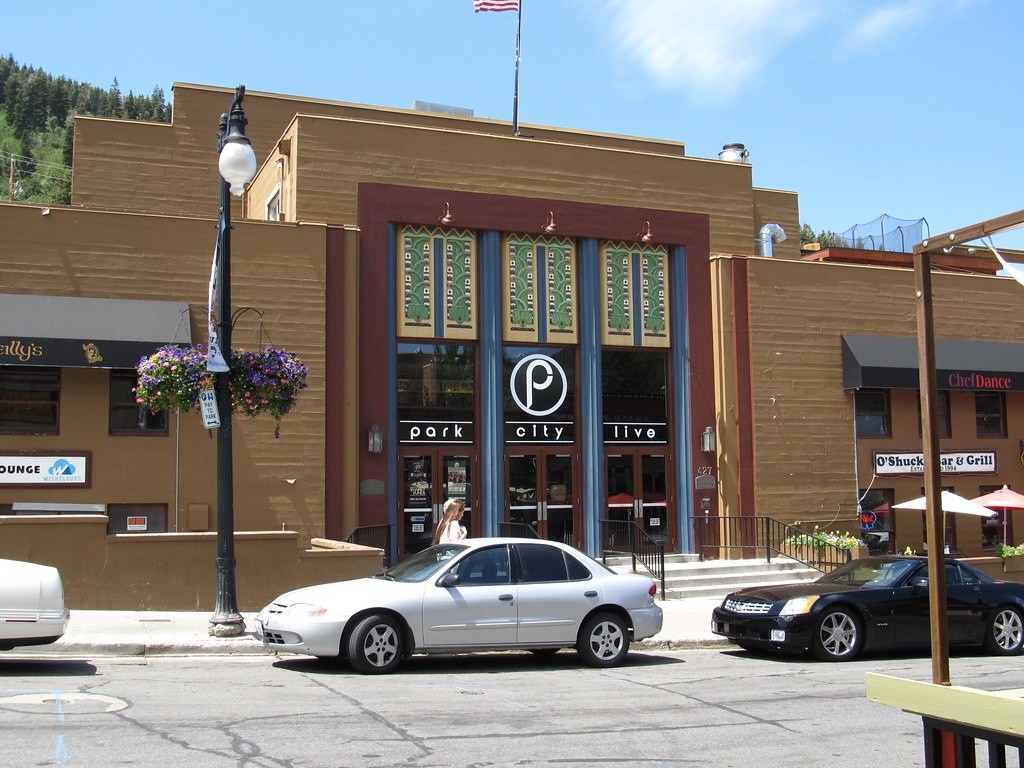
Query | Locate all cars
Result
[0,554,70,651]
[257,535,664,674]
[710,555,1024,664]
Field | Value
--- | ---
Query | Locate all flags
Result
[474,0,519,13]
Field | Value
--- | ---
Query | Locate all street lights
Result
[206,82,258,639]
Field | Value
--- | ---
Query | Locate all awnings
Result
[841,333,1024,393]
[0,293,193,371]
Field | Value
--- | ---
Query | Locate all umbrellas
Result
[968,484,1024,546]
[891,491,996,558]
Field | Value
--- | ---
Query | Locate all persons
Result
[436,499,468,576]
